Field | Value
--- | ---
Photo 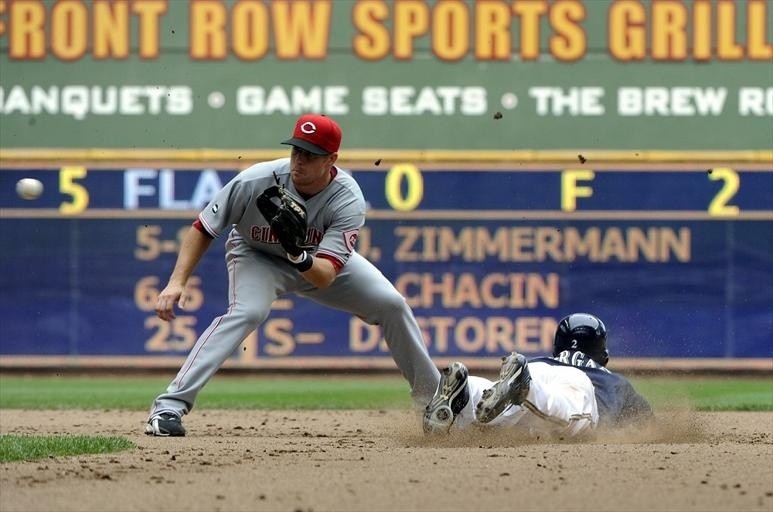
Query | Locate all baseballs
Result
[16,178,43,200]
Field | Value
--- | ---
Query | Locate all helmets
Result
[554,313,610,366]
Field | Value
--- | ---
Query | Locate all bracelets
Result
[285,251,314,275]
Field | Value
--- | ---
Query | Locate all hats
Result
[280,113,342,155]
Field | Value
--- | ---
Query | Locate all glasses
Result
[286,145,331,163]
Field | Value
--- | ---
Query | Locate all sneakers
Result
[474,351,532,424]
[421,360,470,436]
[144,413,186,438]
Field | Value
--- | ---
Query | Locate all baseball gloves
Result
[256,186,308,256]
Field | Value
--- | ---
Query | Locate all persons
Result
[419,309,658,440]
[144,109,444,439]
